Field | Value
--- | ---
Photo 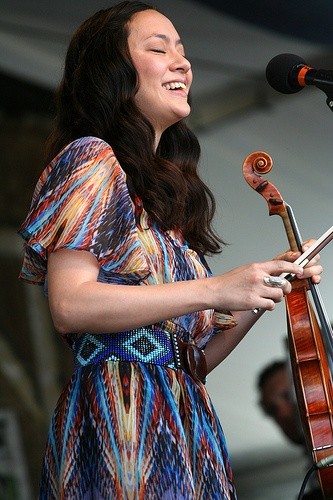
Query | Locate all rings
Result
[262,275,289,289]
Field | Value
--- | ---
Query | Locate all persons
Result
[18,0,324,500]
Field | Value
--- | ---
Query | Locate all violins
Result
[242,150,333,500]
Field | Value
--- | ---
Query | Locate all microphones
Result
[264,54,333,94]
